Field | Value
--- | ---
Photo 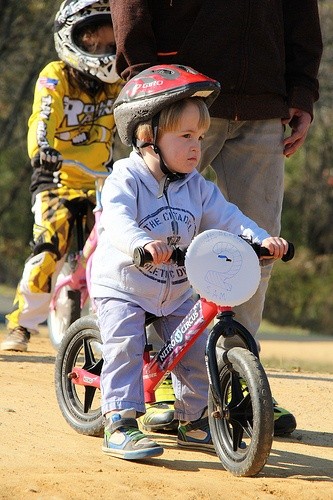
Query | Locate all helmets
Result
[52,0,121,84]
[112,62,222,147]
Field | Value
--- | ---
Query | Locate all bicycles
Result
[31,154,115,354]
[54,227,296,480]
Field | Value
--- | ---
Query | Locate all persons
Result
[86,63,290,460]
[1,0,130,354]
[108,0,324,435]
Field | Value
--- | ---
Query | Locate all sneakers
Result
[1,325,31,351]
[136,368,180,432]
[223,374,297,436]
[100,406,164,460]
[177,406,247,454]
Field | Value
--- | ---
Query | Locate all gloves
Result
[32,148,63,173]
